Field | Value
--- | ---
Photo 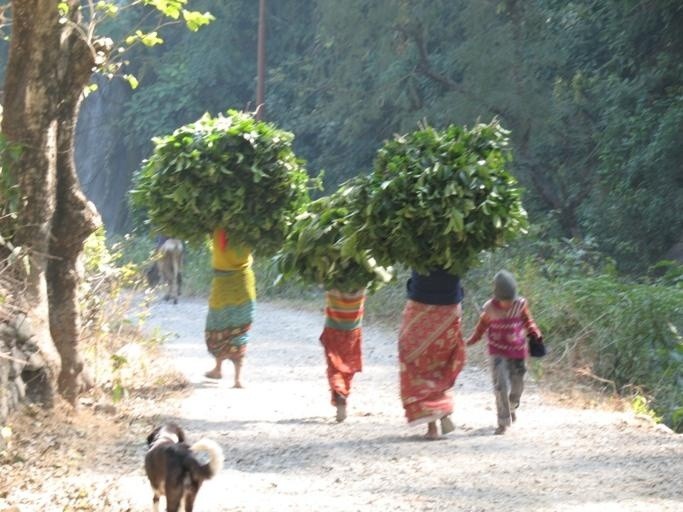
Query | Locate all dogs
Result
[144,421,224,512]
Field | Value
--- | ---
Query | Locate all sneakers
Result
[495,410,517,435]
[423,415,457,439]
[336,395,348,422]
[205,371,222,378]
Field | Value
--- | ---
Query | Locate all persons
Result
[319,280,365,422]
[464,270,543,435]
[204,227,256,388]
[396,267,468,438]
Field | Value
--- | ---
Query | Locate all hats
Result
[493,270,517,301]
